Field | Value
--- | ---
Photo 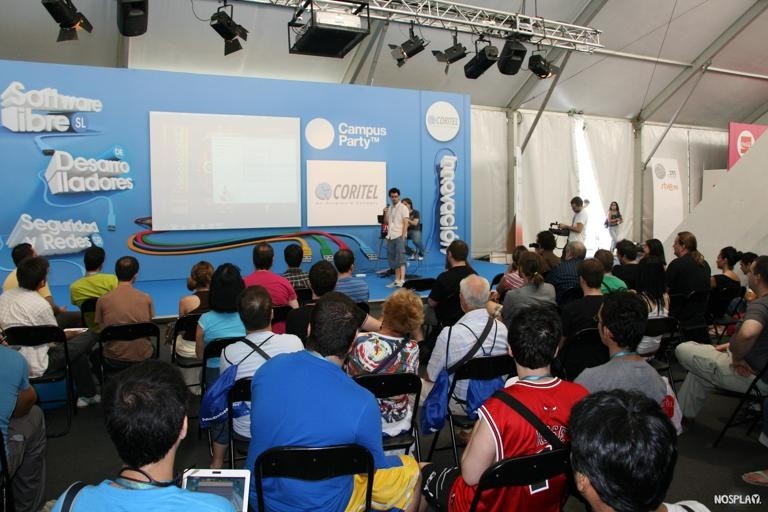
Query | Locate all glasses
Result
[593,313,602,324]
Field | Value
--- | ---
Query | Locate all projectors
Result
[296,12,361,55]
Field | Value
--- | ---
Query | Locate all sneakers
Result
[77,394,102,408]
[395,280,405,287]
[385,281,397,288]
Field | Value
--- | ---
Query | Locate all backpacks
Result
[460,318,506,418]
[421,370,450,434]
[193,334,271,428]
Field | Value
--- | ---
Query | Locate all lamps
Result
[40,0,551,78]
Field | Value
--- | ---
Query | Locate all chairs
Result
[0,274,768,512]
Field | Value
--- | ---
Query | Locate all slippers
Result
[741,470,768,487]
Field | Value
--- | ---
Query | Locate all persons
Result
[242,292,423,512]
[1,231,759,410]
[382,187,410,288]
[209,284,304,470]
[0,335,57,512]
[418,305,591,512]
[740,397,768,487]
[676,256,768,436]
[401,197,425,261]
[417,274,508,447]
[567,388,711,512]
[608,201,623,252]
[343,287,424,457]
[559,196,589,259]
[51,361,240,512]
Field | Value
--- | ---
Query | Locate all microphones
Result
[384,204,390,214]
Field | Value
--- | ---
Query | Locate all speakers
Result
[498,42,527,76]
[117,0,149,36]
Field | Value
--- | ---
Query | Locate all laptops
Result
[182,469,251,512]
